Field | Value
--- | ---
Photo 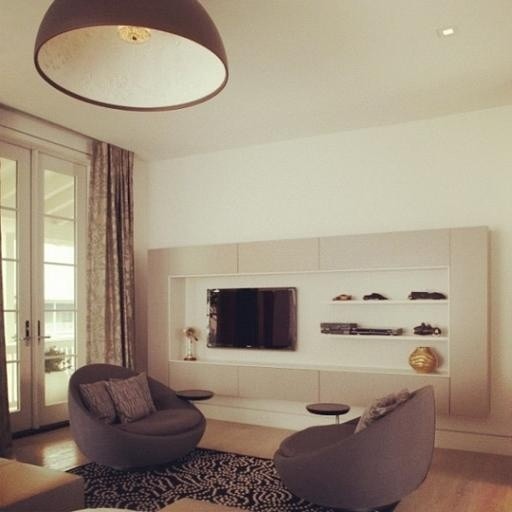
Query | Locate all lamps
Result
[34,0,231,111]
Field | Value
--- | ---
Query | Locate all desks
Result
[305,402,351,424]
[156,497,256,512]
[175,389,216,405]
[0,458,84,512]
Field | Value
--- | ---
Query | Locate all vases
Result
[408,346,438,373]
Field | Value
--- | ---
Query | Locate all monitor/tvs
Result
[207,287,297,351]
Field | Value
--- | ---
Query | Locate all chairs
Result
[274,384,437,512]
[67,363,207,470]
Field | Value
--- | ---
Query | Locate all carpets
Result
[64,448,397,512]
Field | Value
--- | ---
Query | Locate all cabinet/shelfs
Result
[316,297,451,375]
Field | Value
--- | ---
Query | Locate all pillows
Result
[78,380,117,425]
[354,388,410,433]
[105,371,157,425]
